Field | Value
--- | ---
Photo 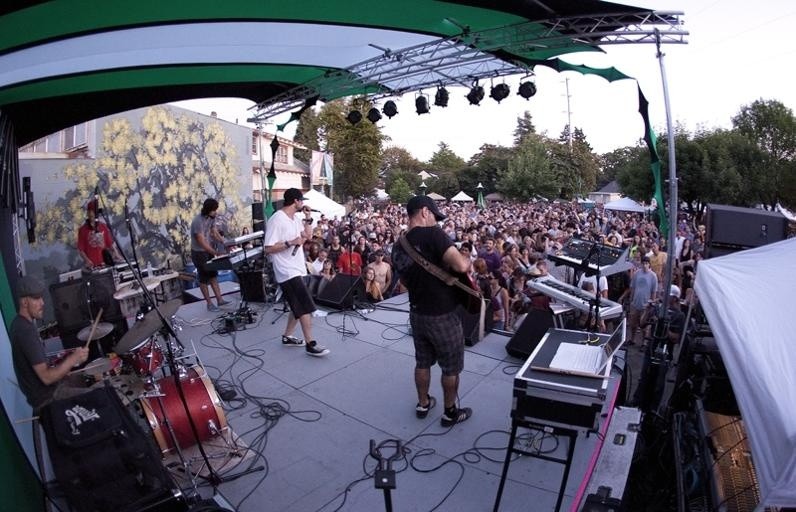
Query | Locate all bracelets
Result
[284,239,291,249]
[302,217,314,226]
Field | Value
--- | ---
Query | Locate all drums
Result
[129,337,165,378]
[138,363,228,455]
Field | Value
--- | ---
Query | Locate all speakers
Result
[47,271,128,368]
[464,298,493,346]
[39,384,167,512]
[505,308,575,361]
[696,203,789,324]
[316,272,366,310]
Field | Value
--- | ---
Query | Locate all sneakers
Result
[306,340,330,357]
[415,396,436,419]
[441,407,473,427]
[217,300,233,307]
[281,335,307,347]
[207,303,221,312]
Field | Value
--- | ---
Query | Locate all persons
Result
[391,194,473,425]
[75,200,126,268]
[260,186,331,359]
[626,283,687,412]
[5,273,108,407]
[187,198,239,312]
[242,193,704,361]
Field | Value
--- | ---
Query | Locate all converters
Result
[225,317,236,328]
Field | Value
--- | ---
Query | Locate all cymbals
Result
[77,322,114,341]
[86,358,114,375]
[118,299,180,352]
[114,279,160,299]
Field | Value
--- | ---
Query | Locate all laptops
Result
[549,317,627,375]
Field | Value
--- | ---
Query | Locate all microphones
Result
[125,200,129,228]
[292,243,300,256]
[580,240,598,271]
[94,187,99,234]
[303,207,320,212]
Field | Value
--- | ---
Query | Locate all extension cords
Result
[218,322,245,334]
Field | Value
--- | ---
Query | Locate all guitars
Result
[452,270,481,315]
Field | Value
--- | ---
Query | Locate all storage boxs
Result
[510,324,616,434]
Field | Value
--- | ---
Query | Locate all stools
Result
[28,406,60,511]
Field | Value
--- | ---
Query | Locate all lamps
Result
[344,68,538,126]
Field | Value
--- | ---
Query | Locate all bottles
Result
[146,261,154,281]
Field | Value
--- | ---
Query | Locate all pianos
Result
[204,230,265,270]
[527,273,622,321]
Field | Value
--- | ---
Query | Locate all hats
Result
[375,249,384,257]
[666,284,682,299]
[408,195,446,221]
[16,276,48,298]
[284,188,310,201]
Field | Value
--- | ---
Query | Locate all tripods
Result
[328,219,377,321]
[144,373,242,498]
[99,206,265,499]
[271,284,291,324]
